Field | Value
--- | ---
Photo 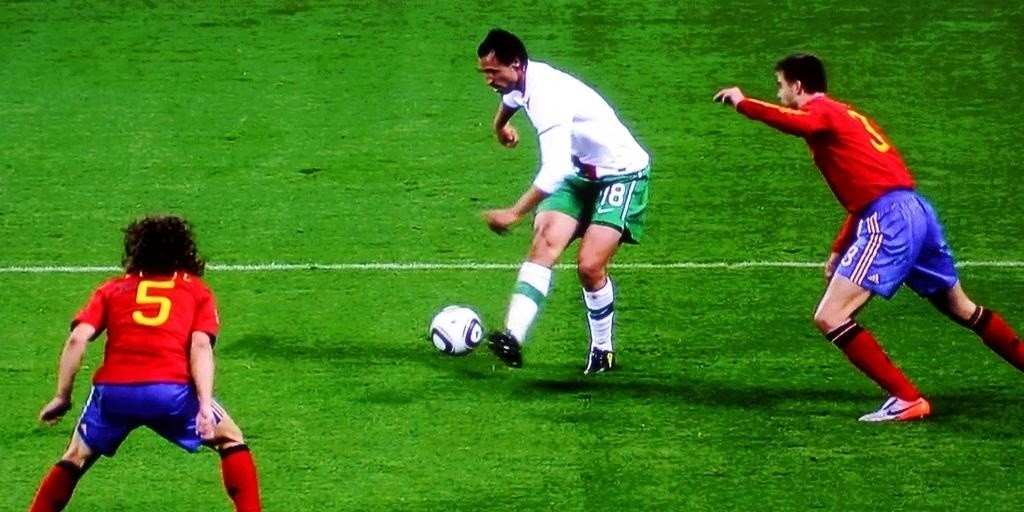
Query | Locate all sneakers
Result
[486,328,524,370]
[854,393,932,426]
[582,347,615,379]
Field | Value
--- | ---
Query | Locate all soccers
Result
[428,304,484,356]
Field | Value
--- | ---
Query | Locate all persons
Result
[29,214,261,512]
[477,28,651,375]
[712,54,1024,422]
